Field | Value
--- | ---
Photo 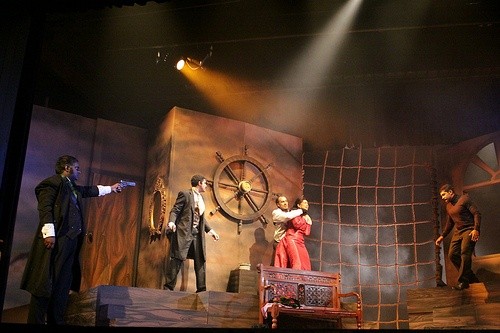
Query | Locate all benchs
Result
[255,264,363,330]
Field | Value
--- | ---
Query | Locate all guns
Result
[116,180,136,192]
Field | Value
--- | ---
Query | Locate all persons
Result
[19,155,121,326]
[270,195,308,267]
[162,175,219,293]
[274,197,312,270]
[435,184,481,290]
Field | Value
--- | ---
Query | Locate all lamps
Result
[164,53,186,70]
